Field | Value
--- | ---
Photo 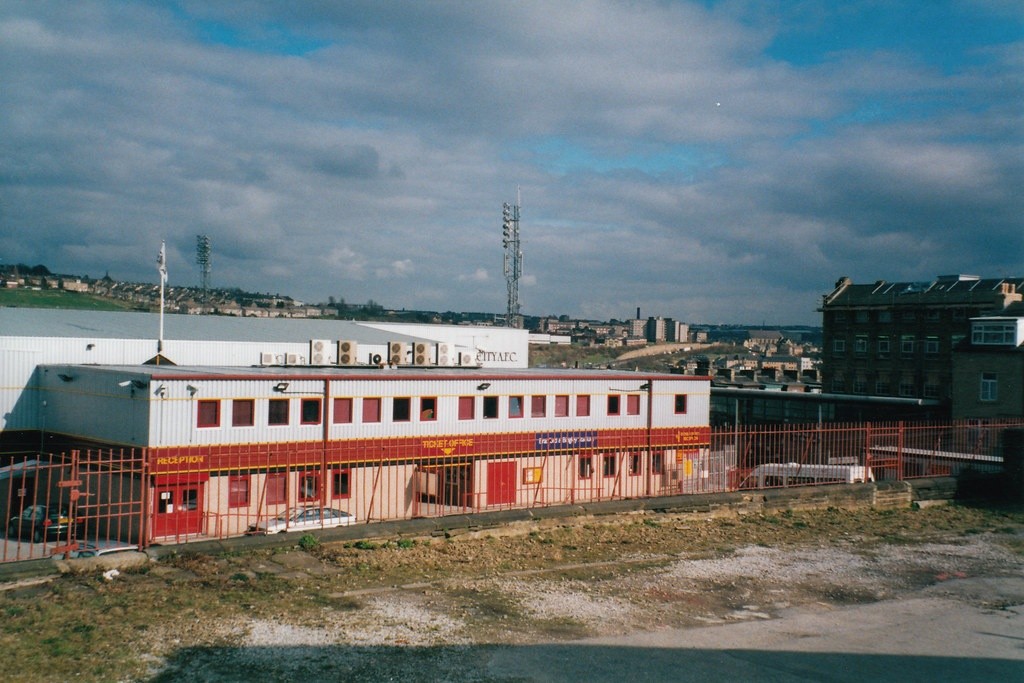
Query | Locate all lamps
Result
[478,383,490,390]
[276,383,289,390]
[58,375,72,381]
[132,380,145,388]
[641,384,650,389]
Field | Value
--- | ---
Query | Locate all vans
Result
[738,463,874,492]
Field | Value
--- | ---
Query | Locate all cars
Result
[244,505,355,535]
[8,504,85,544]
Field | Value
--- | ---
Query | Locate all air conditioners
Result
[285,353,301,365]
[338,340,356,366]
[437,342,455,366]
[261,352,275,366]
[414,342,432,365]
[389,341,407,364]
[460,352,475,366]
[311,339,331,365]
[370,353,387,365]
